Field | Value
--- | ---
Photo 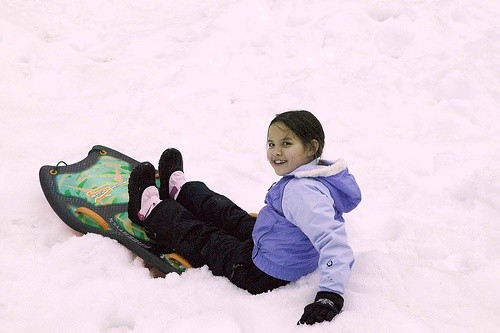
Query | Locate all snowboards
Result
[39,145,259,278]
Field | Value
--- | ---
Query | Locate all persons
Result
[128,110,361,325]
